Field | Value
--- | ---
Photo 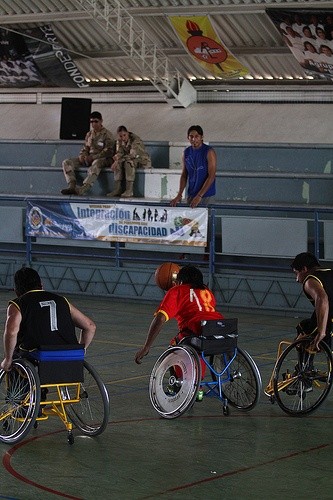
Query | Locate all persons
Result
[1,267,97,418]
[107,125,151,198]
[134,265,225,402]
[169,125,217,267]
[60,111,116,195]
[285,252,333,391]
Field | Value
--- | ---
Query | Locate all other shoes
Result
[195,390,204,401]
[11,406,43,418]
[287,382,312,392]
[165,384,176,396]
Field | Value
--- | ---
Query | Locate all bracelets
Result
[197,194,202,198]
[178,194,182,197]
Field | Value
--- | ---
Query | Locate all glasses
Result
[90,120,100,123]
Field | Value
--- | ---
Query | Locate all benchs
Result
[0,137,333,215]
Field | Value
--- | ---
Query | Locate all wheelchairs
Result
[0,343,110,444]
[149,318,263,420]
[263,319,333,417]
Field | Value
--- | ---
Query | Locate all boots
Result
[76,183,91,196]
[111,180,123,197]
[121,181,134,198]
[61,181,76,195]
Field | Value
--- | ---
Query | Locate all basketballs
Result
[155,263,180,292]
